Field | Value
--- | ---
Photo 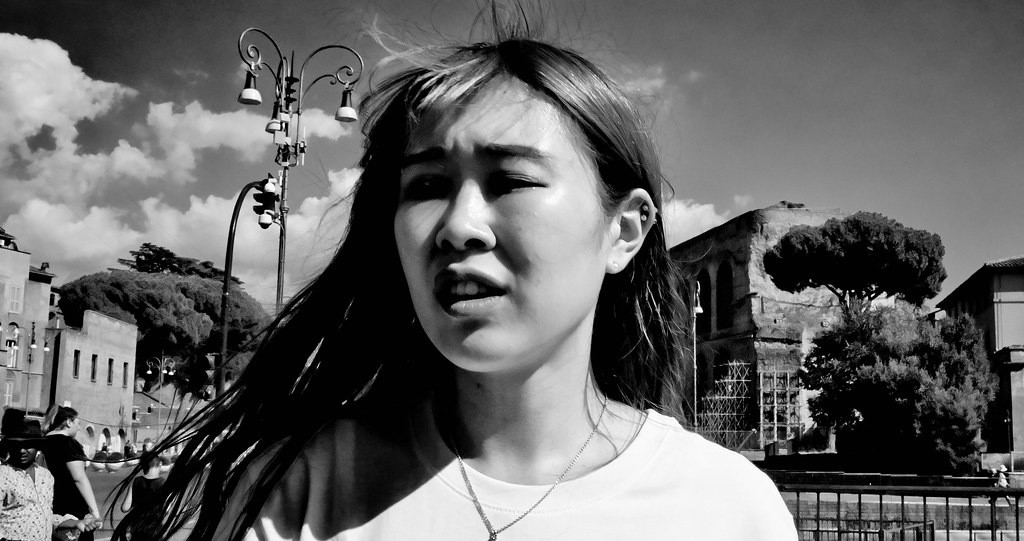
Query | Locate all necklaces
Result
[428,385,608,540]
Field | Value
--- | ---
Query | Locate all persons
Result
[988,465,1014,507]
[121,451,170,540]
[0,419,102,541]
[35,404,103,540]
[104,33,800,540]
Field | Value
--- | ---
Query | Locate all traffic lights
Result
[253,185,275,215]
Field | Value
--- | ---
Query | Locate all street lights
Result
[146,351,176,446]
[1004,409,1014,472]
[13,322,50,415]
[237,27,364,315]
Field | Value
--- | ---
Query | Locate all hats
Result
[2,420,46,442]
